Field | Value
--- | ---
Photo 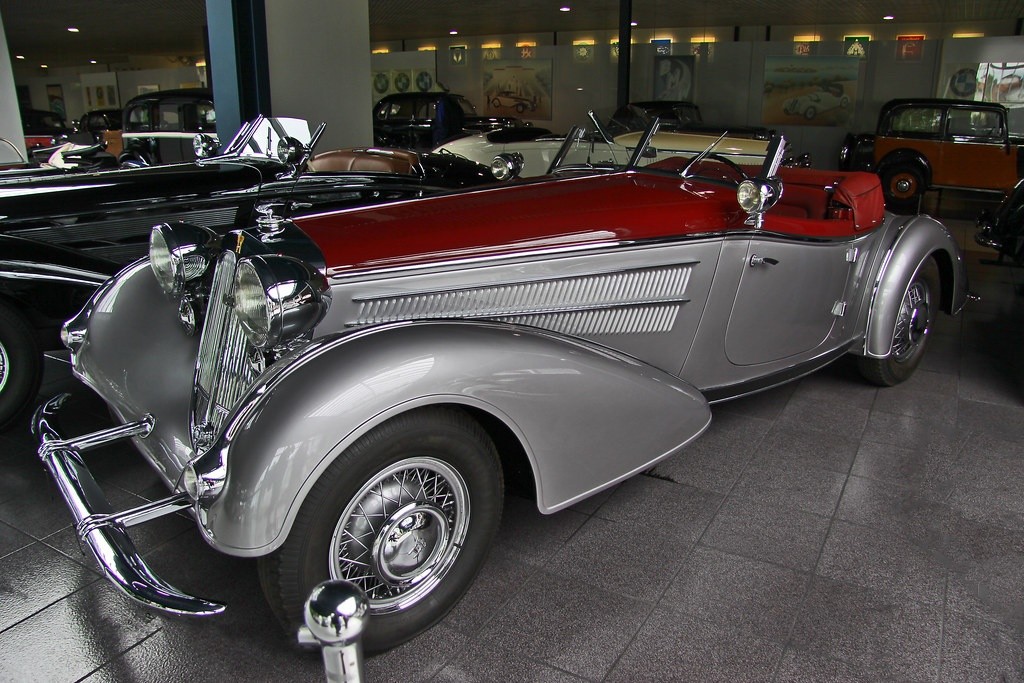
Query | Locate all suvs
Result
[839,97,1024,216]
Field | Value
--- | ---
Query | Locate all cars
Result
[432,110,649,179]
[25,115,972,660]
[0,90,219,186]
[0,115,520,426]
[374,93,525,153]
[600,99,777,170]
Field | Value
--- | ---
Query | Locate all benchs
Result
[643,156,830,220]
[307,147,418,174]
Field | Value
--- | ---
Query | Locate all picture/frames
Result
[412,66,435,92]
[371,67,391,103]
[654,55,695,102]
[761,55,859,127]
[392,68,412,94]
[482,59,552,121]
